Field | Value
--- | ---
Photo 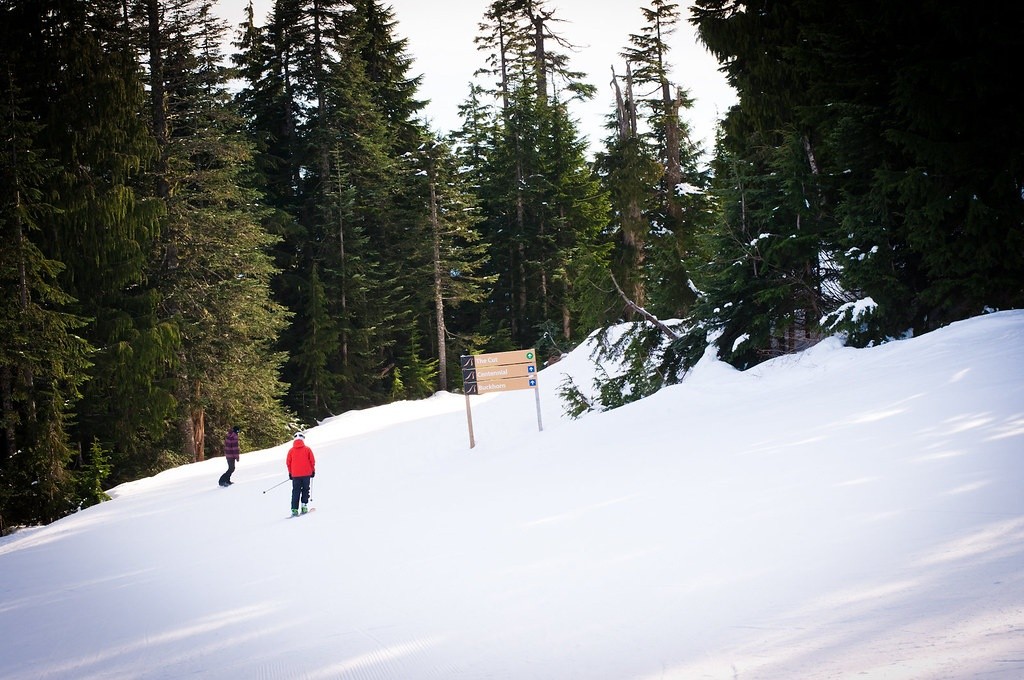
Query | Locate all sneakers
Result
[301,503,308,514]
[291,509,298,516]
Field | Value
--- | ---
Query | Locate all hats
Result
[294,432,305,441]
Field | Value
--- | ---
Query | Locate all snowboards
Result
[285,508,318,519]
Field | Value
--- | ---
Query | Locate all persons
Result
[219,426,240,487]
[286,431,316,515]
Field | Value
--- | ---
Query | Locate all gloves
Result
[311,472,315,478]
[289,473,292,480]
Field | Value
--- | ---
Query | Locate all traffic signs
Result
[458,349,539,394]
